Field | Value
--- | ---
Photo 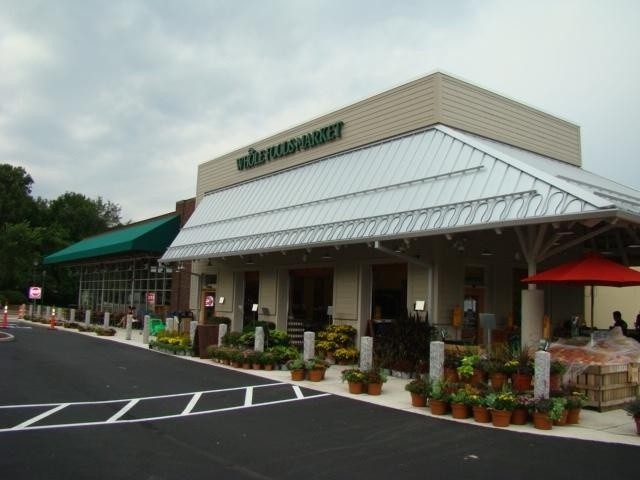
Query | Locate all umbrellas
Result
[520,250,640,333]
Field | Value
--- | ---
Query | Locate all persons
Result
[132,307,141,329]
[609,310,627,333]
[116,306,132,328]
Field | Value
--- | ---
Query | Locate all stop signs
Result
[29,287,42,300]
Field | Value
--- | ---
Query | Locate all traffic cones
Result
[0,305,10,329]
[17,305,24,319]
[48,308,57,330]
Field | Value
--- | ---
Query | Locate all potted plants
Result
[207,343,281,372]
[149,329,193,356]
[623,398,640,435]
[340,367,388,396]
[404,340,586,431]
[287,357,331,382]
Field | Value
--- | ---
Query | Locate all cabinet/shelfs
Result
[544,362,640,413]
